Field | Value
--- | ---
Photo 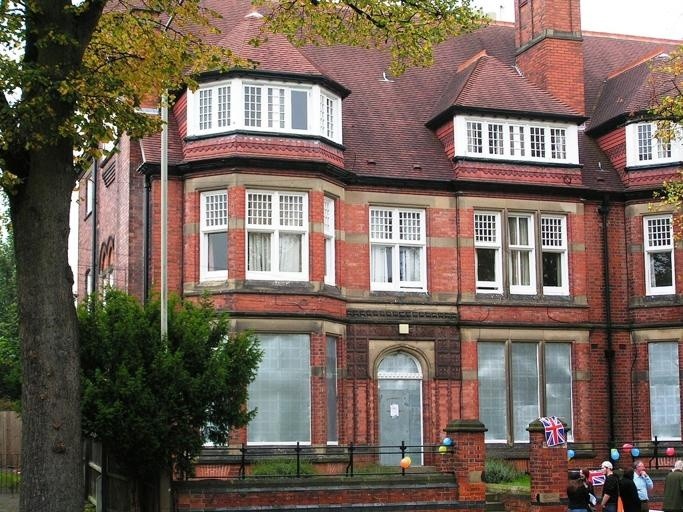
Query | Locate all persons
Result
[662,460,683,512]
[600,461,618,512]
[613,466,625,512]
[566,474,590,512]
[632,460,654,512]
[619,467,641,512]
[579,468,594,512]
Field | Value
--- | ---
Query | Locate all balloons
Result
[568,450,574,457]
[438,446,446,455]
[611,451,620,460]
[666,447,674,456]
[443,438,452,446]
[622,444,632,452]
[400,456,411,468]
[631,449,639,456]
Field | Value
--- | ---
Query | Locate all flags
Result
[580,470,606,486]
[539,415,566,447]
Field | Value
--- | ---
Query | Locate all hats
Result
[601,461,613,470]
[671,461,683,473]
